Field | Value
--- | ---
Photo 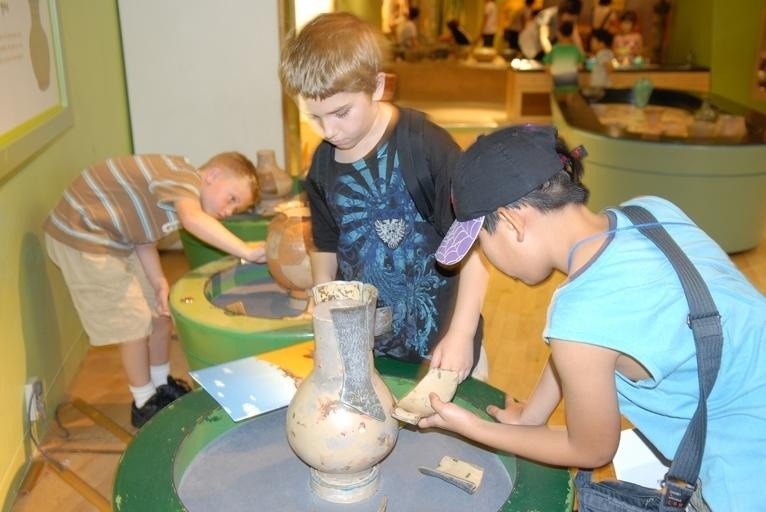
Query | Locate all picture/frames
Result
[0,0,74,179]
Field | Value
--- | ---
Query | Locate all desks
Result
[549,86,766,256]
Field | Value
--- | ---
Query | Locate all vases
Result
[266,200,314,312]
[255,151,294,215]
[286,280,399,504]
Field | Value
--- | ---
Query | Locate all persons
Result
[419,124,766,509]
[282,14,490,377]
[40,153,271,430]
[395,1,670,104]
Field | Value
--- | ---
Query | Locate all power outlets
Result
[25,377,40,422]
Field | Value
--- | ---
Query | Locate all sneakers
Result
[131,376,190,428]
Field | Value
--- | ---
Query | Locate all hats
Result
[433,124,588,267]
